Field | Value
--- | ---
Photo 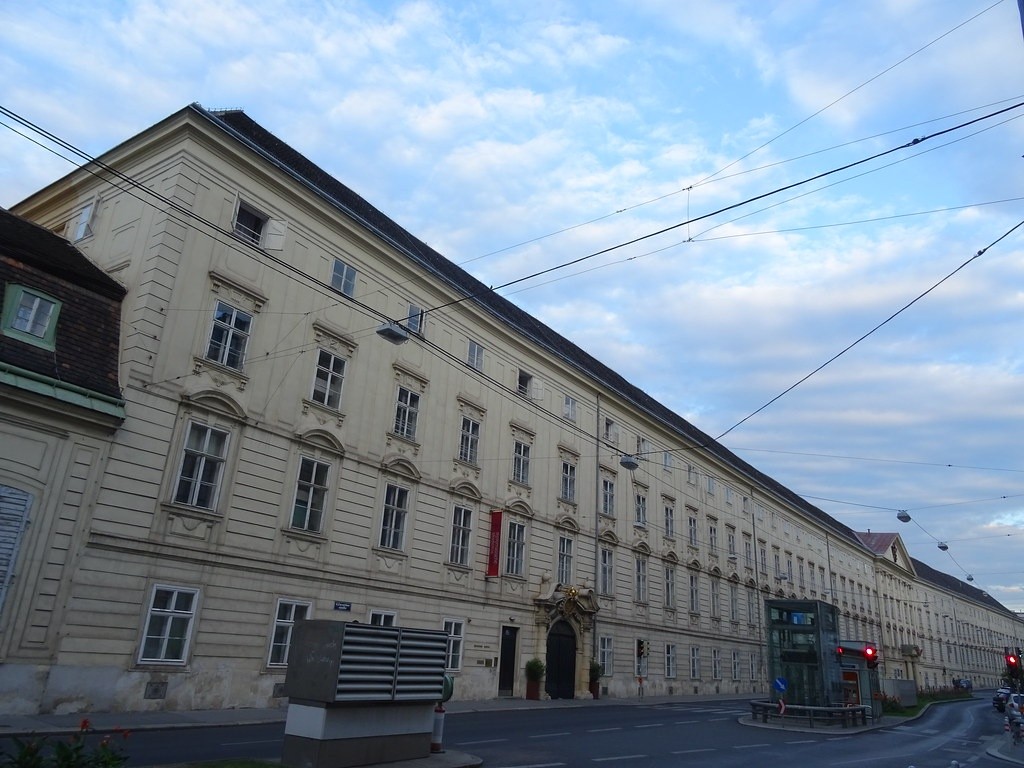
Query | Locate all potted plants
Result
[524,658,547,699]
[588,662,603,699]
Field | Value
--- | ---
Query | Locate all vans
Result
[954,679,973,693]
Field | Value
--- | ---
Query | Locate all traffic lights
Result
[1006,653,1021,680]
[865,647,879,670]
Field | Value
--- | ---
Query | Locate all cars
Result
[1004,693,1024,725]
[992,689,1011,713]
